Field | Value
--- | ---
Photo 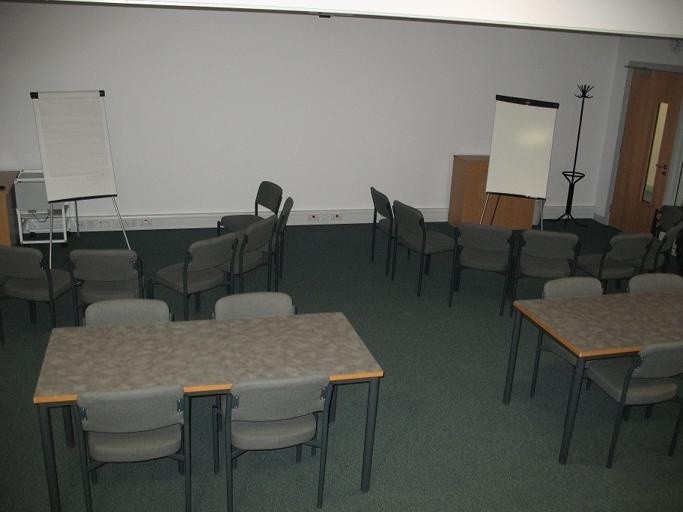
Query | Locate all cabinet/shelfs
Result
[13,170,68,270]
[1,171,20,247]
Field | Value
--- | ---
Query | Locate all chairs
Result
[84,298,172,327]
[211,376,332,511]
[584,342,682,468]
[212,292,296,320]
[72,384,192,512]
[530,277,603,398]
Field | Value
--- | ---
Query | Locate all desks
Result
[504,288,683,465]
[448,156,535,230]
[32,312,384,512]
